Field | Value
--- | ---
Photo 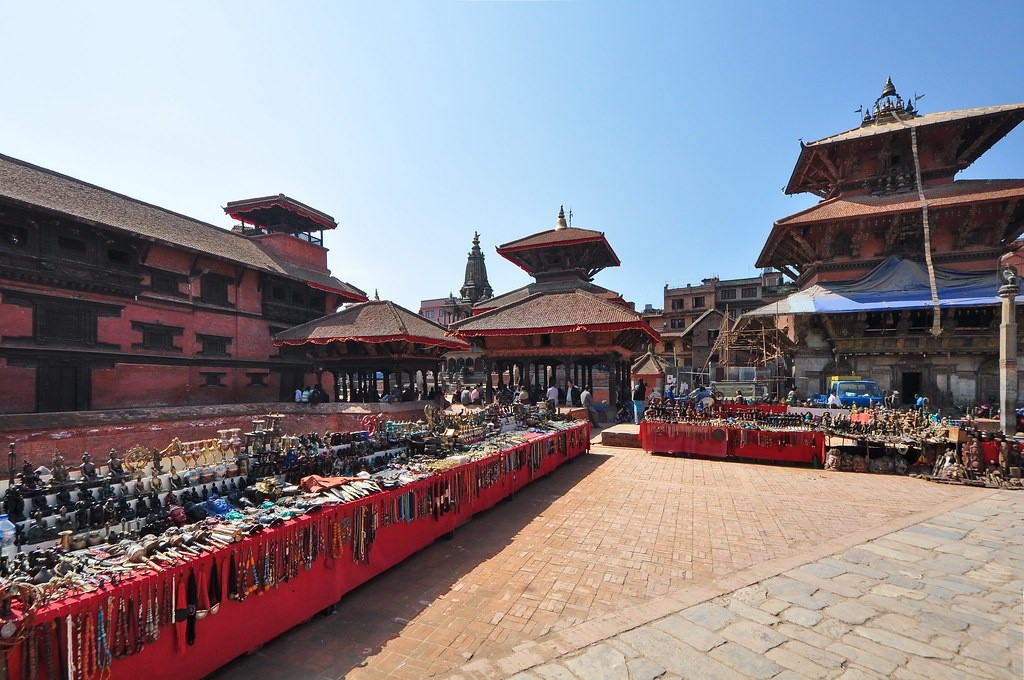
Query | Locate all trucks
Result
[814,380,885,409]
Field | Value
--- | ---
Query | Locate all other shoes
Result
[594,425,602,428]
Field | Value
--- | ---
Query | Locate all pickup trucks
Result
[674,386,711,410]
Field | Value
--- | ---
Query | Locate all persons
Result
[544,382,559,406]
[665,384,675,399]
[414,389,422,401]
[884,396,892,409]
[563,380,577,407]
[3,402,521,545]
[734,390,742,404]
[823,398,952,439]
[786,386,798,407]
[494,383,529,405]
[632,377,646,425]
[428,387,435,400]
[889,391,899,409]
[392,384,400,400]
[451,382,484,404]
[580,384,601,428]
[294,384,320,403]
[914,394,923,407]
[694,386,714,402]
[828,390,842,408]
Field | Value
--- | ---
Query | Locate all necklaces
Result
[21,425,585,680]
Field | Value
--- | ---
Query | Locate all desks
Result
[0,408,592,680]
[639,404,1024,468]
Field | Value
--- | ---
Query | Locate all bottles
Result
[0,514,17,563]
